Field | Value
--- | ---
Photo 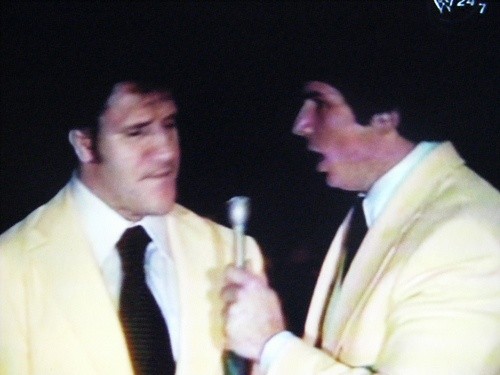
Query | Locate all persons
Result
[0,55,271,375]
[218,33,500,375]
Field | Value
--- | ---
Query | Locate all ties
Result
[343,196,369,290]
[115,227,177,375]
